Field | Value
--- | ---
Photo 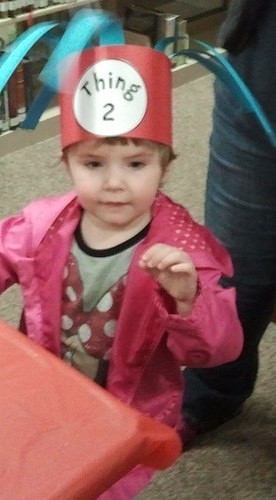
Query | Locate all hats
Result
[0,9,274,160]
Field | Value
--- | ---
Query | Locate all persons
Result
[0,47,243,500]
[174,0,276,446]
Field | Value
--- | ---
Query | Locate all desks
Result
[0,0,98,131]
[0,316,182,499]
